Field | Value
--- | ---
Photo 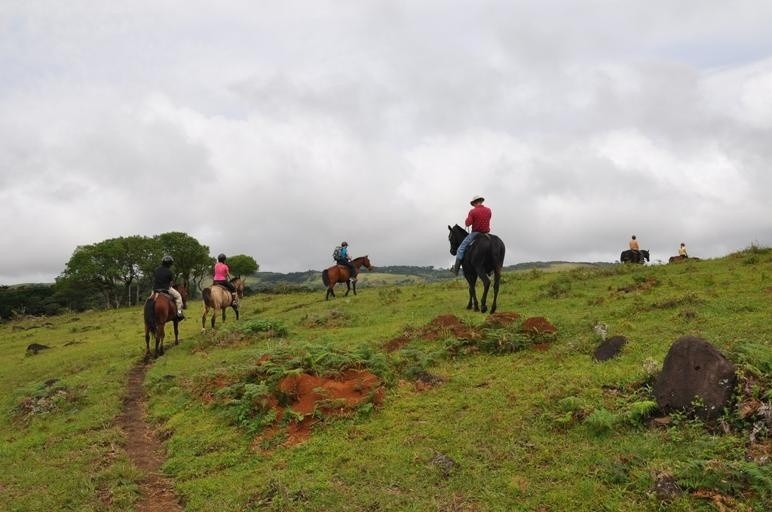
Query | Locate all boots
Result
[231,293,238,307]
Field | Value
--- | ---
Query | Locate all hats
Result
[341,242,348,246]
[470,195,484,206]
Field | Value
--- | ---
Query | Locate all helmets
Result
[218,254,226,262]
[161,256,174,265]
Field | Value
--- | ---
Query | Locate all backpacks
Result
[333,246,343,261]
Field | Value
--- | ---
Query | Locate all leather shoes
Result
[177,313,184,321]
[450,268,458,276]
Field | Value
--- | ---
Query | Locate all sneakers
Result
[349,277,354,281]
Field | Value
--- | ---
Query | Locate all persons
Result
[152,255,185,321]
[450,194,492,276]
[212,254,239,308]
[629,235,644,264]
[678,242,688,258]
[336,241,357,282]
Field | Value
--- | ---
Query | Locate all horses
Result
[448,223,506,315]
[620,249,649,265]
[143,280,189,359]
[668,256,688,264]
[322,255,372,301]
[202,274,246,337]
